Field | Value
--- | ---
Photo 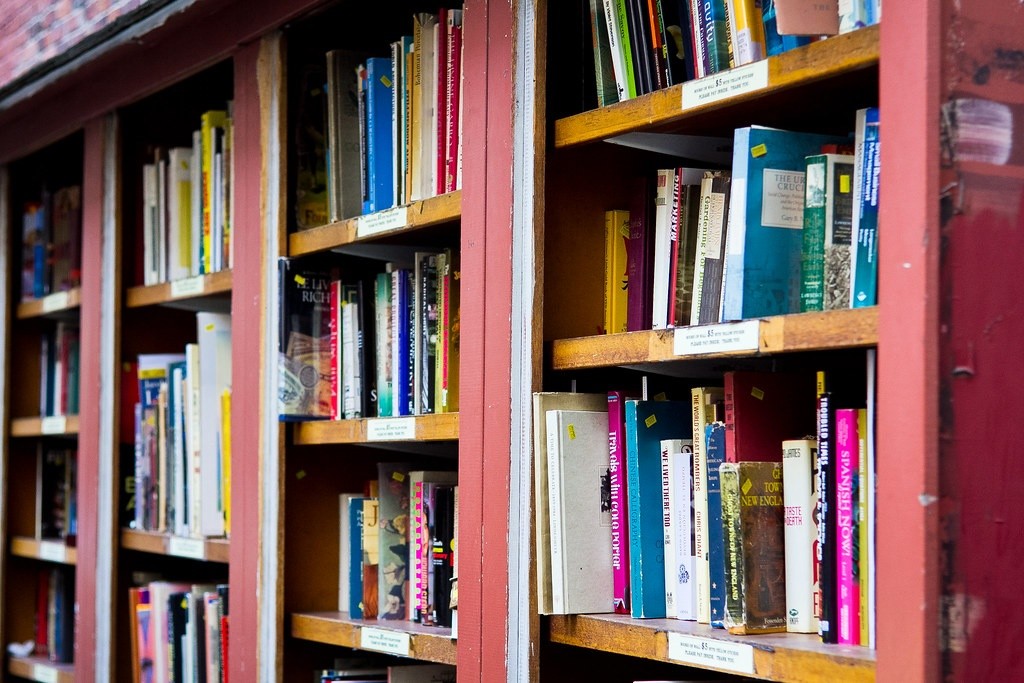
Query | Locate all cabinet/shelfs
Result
[0,0,1024,683]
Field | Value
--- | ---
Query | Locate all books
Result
[0,0,880,683]
[851,106,878,307]
